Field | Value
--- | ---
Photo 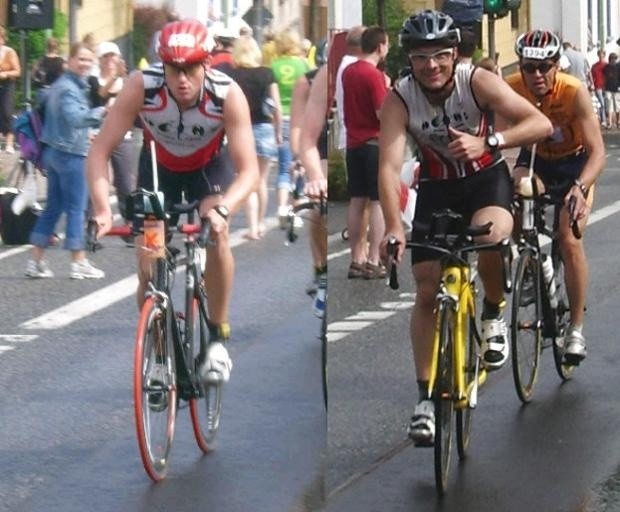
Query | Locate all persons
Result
[493,30,608,363]
[376,8,553,442]
[343,26,392,281]
[561,40,619,128]
[0,21,328,319]
[336,25,368,151]
[84,21,260,414]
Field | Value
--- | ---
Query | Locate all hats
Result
[98,41,119,58]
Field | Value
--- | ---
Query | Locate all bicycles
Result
[285,184,327,416]
[505,182,586,402]
[84,194,231,484]
[383,209,512,499]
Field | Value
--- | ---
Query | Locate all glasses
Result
[522,62,553,73]
[409,48,453,62]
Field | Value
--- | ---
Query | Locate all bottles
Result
[144,213,167,260]
[519,177,534,230]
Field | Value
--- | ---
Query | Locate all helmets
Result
[159,19,214,65]
[401,9,461,47]
[515,30,560,61]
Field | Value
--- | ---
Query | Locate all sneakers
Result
[71,260,105,279]
[313,273,326,318]
[25,260,53,277]
[407,400,435,443]
[277,205,303,228]
[149,357,171,407]
[480,313,509,366]
[201,342,233,382]
[564,331,586,360]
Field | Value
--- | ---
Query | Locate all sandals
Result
[348,262,388,278]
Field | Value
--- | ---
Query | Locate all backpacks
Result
[14,97,46,159]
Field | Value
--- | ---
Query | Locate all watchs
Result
[213,203,230,223]
[485,132,500,156]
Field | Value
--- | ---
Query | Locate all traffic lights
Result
[483,1,507,15]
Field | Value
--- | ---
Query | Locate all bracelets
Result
[573,179,588,196]
